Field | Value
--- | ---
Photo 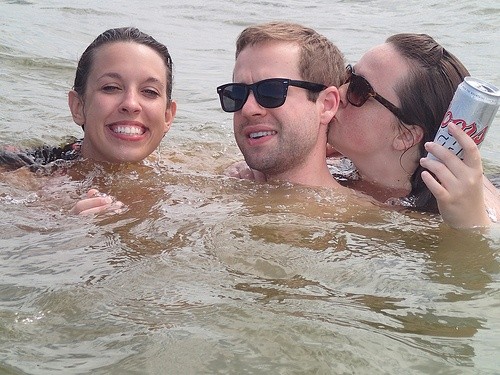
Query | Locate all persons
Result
[0,29,177,218]
[216,21,500,229]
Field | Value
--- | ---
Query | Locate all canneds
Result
[424,76,500,164]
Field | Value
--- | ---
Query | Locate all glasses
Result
[339,64,403,120]
[217,77,328,112]
